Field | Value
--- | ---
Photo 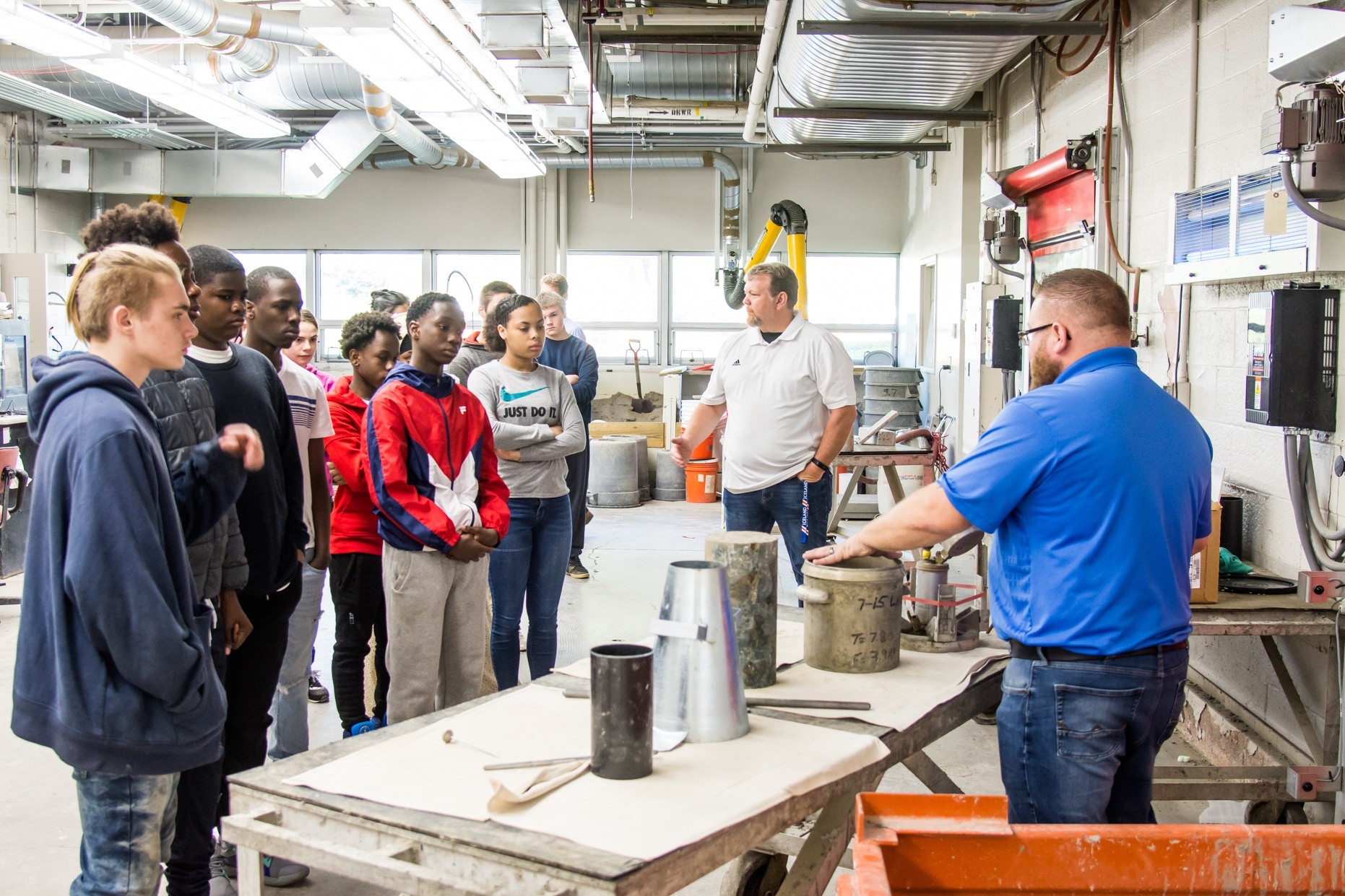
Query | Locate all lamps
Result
[299,5,483,112]
[413,98,547,179]
[60,45,292,140]
[0,0,127,59]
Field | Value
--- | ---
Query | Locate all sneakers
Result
[220,838,310,887]
[209,836,237,896]
[565,556,590,578]
[307,670,329,704]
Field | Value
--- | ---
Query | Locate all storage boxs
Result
[1186,500,1224,605]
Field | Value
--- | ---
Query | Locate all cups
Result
[914,560,950,625]
[589,644,654,781]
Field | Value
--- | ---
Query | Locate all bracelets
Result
[810,456,829,474]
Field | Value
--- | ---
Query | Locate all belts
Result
[1008,638,1187,661]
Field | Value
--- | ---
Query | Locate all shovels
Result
[629,338,654,414]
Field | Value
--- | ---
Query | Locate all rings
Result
[828,546,836,555]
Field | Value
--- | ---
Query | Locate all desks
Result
[1151,558,1345,825]
[216,601,1014,896]
[825,434,936,584]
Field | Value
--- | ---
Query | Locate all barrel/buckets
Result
[714,412,727,473]
[652,450,686,501]
[795,555,905,673]
[684,458,719,503]
[586,434,652,509]
[680,400,715,460]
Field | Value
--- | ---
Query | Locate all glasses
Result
[1018,324,1071,345]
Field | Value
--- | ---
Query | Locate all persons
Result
[534,272,597,580]
[803,269,1213,823]
[327,290,414,741]
[361,293,512,728]
[467,294,587,689]
[445,279,528,651]
[10,243,265,896]
[671,263,857,608]
[80,201,333,896]
